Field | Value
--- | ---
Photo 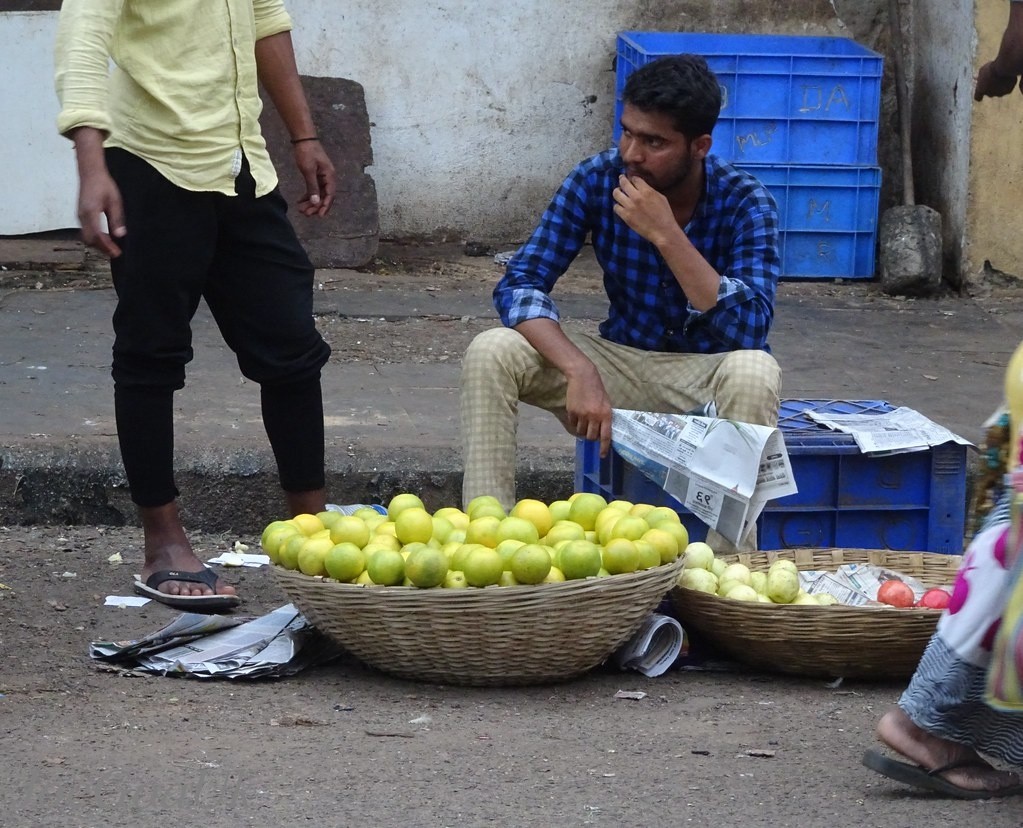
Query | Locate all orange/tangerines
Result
[261,492,687,589]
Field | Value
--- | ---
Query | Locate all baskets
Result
[682,543,976,676]
[255,553,682,685]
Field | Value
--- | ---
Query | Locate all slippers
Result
[860,738,1020,801]
[133,564,241,608]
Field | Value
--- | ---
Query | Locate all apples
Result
[681,542,952,608]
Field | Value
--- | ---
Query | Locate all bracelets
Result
[291,136,320,144]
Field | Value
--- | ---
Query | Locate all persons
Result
[462,51,783,556]
[54,0,339,608]
[862,336,1023,801]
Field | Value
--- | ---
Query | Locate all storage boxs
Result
[615,28,886,170]
[573,398,966,555]
[746,167,882,281]
